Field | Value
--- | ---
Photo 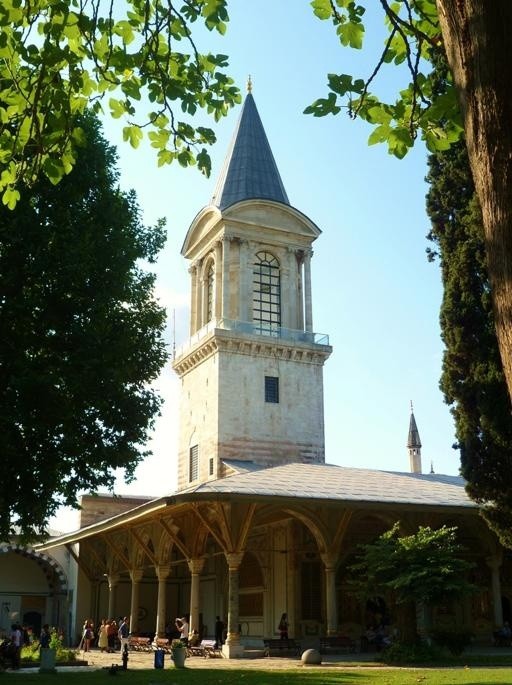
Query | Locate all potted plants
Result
[170,639,188,668]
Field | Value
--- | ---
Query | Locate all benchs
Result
[262,639,302,657]
[130,636,170,653]
[190,639,217,657]
[318,636,356,655]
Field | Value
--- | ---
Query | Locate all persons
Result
[215,616,225,649]
[278,613,292,650]
[82,616,130,653]
[9,625,22,671]
[34,624,52,657]
[377,623,385,636]
[362,622,377,643]
[175,617,189,645]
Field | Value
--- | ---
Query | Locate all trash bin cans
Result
[154,650,165,668]
[40,648,56,672]
